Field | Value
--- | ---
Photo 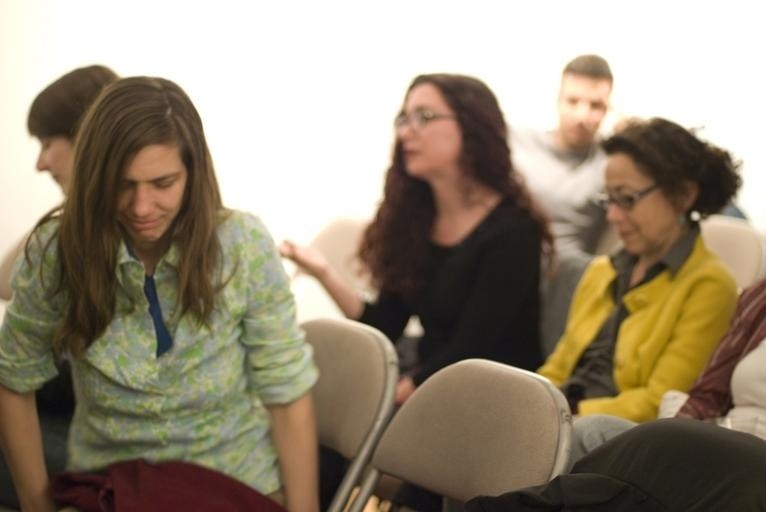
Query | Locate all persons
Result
[465,273,765,511]
[1,66,320,511]
[505,55,613,296]
[532,117,743,475]
[278,73,556,511]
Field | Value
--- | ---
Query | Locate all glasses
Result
[394,110,458,131]
[599,182,665,213]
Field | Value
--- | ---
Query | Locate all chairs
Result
[694,215,765,292]
[299,313,404,509]
[346,356,579,509]
